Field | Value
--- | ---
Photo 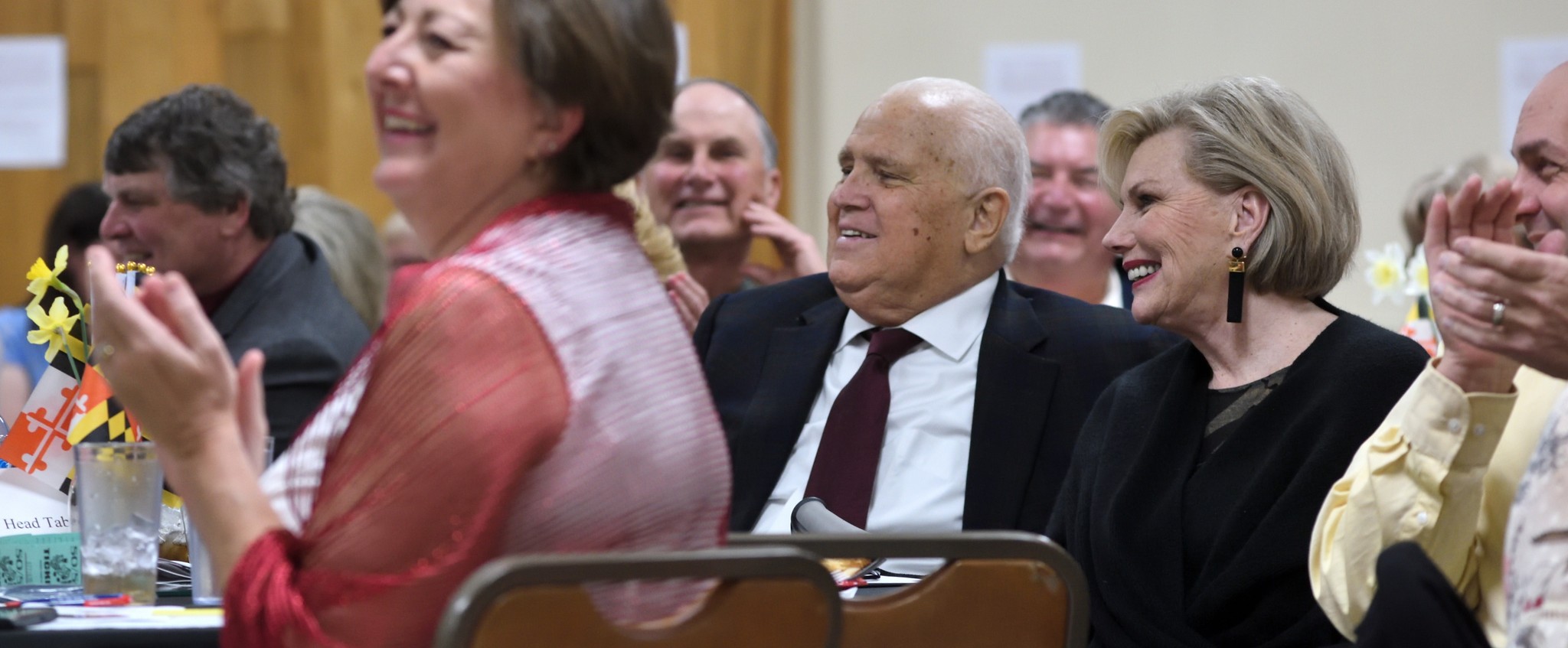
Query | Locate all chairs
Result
[427,541,843,648]
[712,527,1092,646]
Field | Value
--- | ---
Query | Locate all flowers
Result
[1361,242,1446,362]
[22,245,158,445]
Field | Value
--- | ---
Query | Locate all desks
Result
[3,566,237,648]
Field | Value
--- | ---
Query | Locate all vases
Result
[75,439,164,605]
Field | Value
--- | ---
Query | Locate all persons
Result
[0,179,127,436]
[1003,82,1155,322]
[696,69,1170,595]
[95,81,379,500]
[376,206,430,281]
[1022,72,1492,646]
[290,180,387,330]
[1303,58,1568,648]
[69,2,738,648]
[608,176,712,342]
[636,73,830,324]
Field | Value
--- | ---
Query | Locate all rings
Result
[1490,299,1504,326]
[95,339,113,366]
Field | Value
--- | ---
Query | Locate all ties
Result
[803,326,924,528]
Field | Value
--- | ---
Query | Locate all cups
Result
[71,440,166,608]
[182,434,274,606]
